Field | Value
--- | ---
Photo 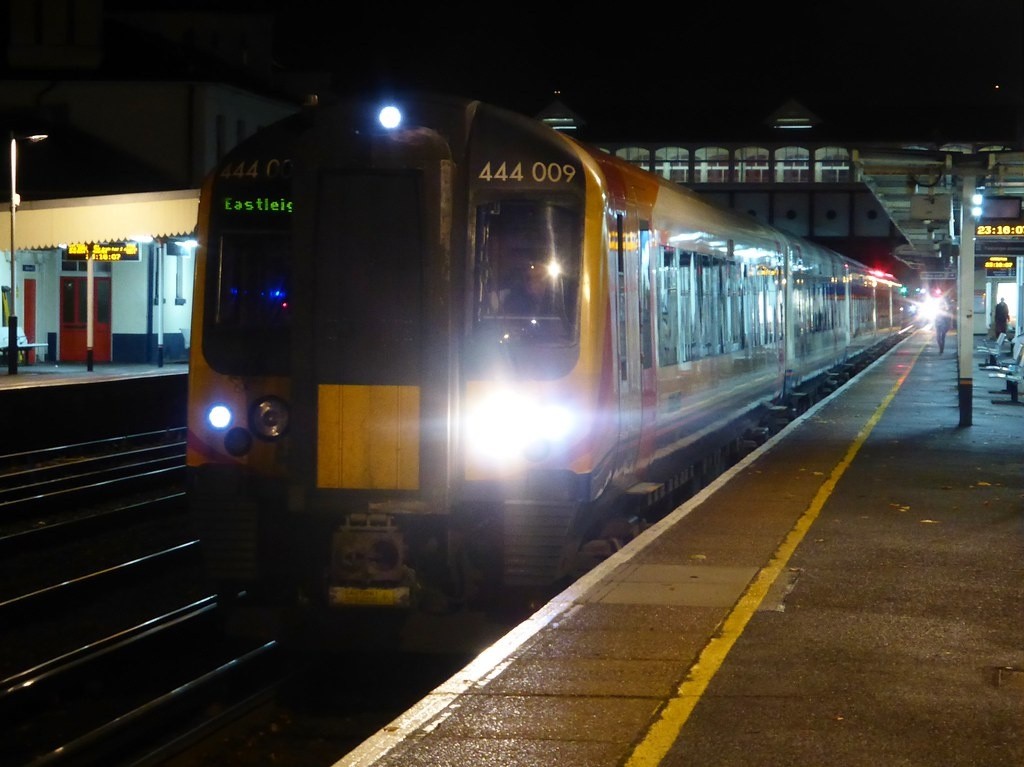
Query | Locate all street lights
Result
[6,128,52,377]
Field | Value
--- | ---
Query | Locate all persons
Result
[934,301,952,357]
[993,297,1012,340]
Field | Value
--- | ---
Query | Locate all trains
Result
[175,92,920,680]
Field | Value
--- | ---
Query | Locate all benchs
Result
[987,352,1023,406]
[0,327,49,365]
[977,332,1006,367]
[986,343,1024,391]
